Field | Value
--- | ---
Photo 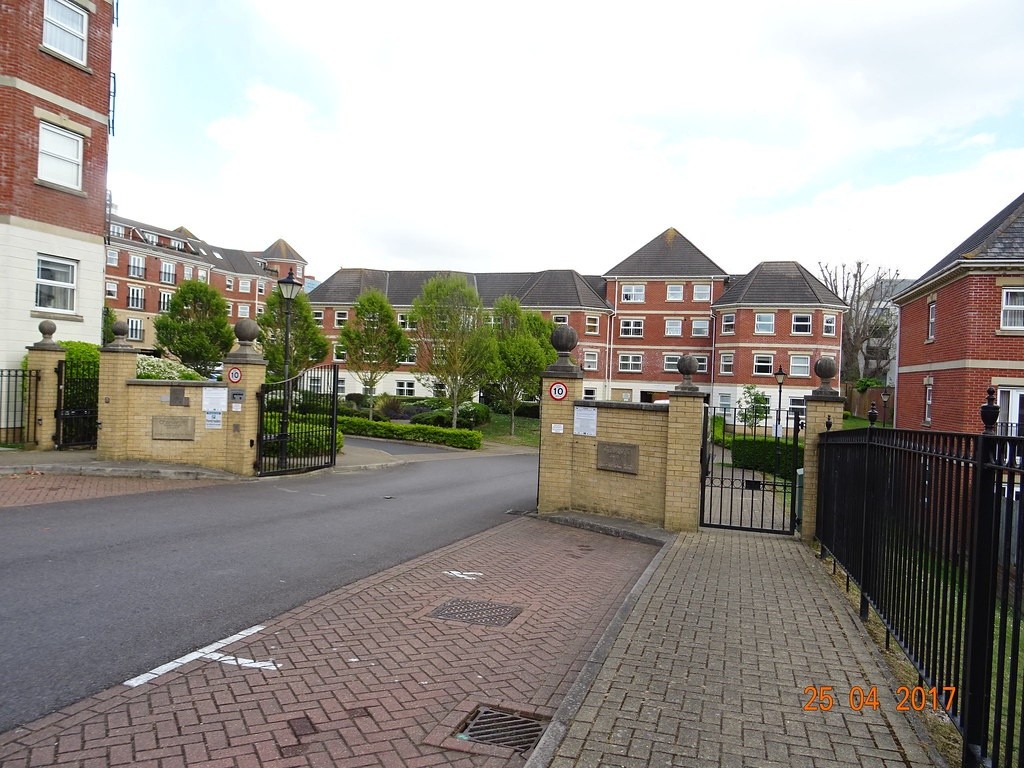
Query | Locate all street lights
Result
[272,264,306,469]
[843,379,851,397]
[880,389,891,429]
[772,365,788,482]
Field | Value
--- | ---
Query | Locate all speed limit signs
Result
[228,367,242,383]
[549,382,567,400]
